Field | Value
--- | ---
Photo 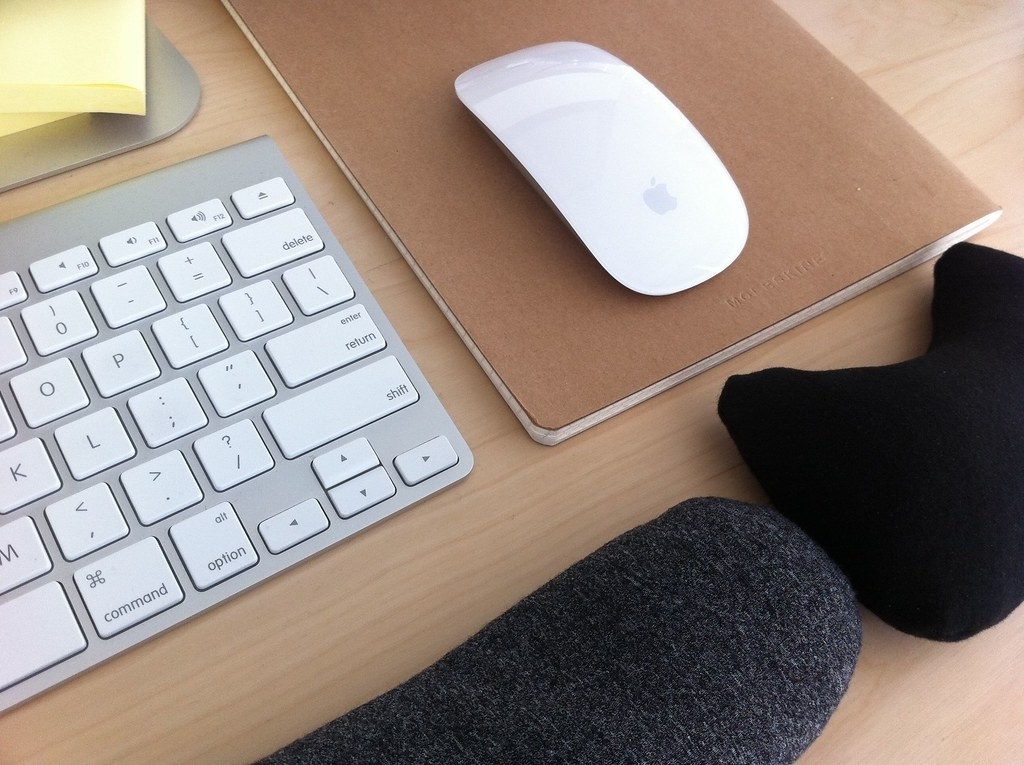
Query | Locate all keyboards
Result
[0,134,474,713]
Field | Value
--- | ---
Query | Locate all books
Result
[215,0,1001,447]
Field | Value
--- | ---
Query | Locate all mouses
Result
[456,42,750,294]
